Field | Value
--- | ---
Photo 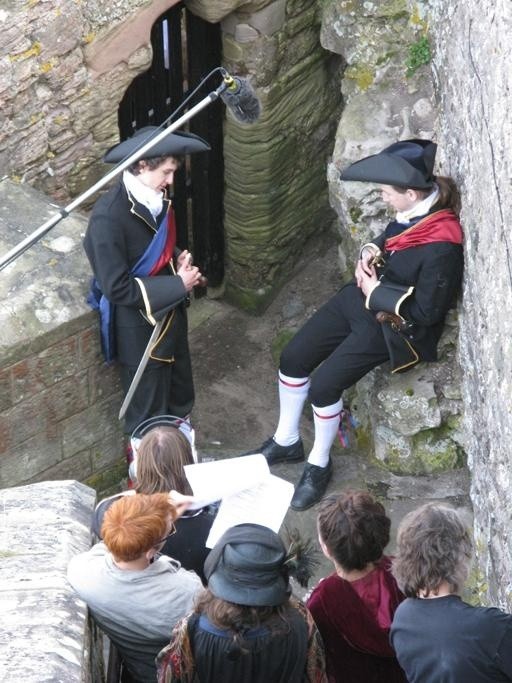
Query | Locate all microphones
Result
[220,75,261,124]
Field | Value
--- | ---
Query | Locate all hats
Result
[204,524,293,607]
[339,139,438,189]
[103,127,210,163]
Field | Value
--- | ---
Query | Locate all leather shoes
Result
[291,454,332,511]
[236,435,305,465]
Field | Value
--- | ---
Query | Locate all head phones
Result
[128,414,198,484]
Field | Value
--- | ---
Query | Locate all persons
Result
[155,522,335,682]
[83,121,203,494]
[92,413,219,587]
[385,500,511,682]
[239,139,464,511]
[67,490,207,682]
[305,487,407,682]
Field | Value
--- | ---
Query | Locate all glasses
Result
[153,521,177,545]
